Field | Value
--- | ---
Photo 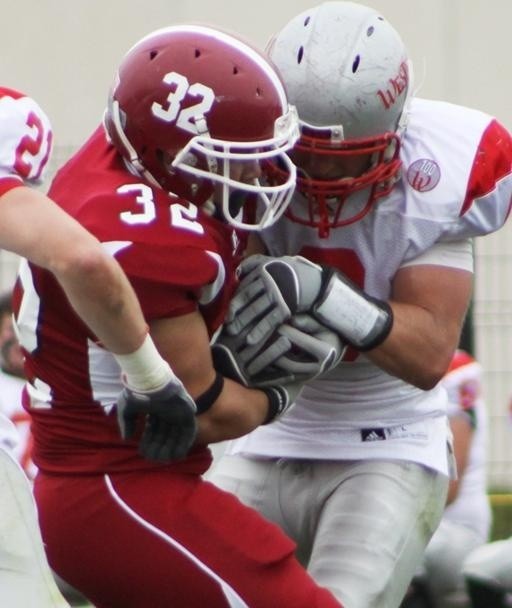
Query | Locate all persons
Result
[462,535,512,608]
[402,348,494,607]
[0,288,46,479]
[1,83,198,608]
[198,1,512,608]
[31,25,347,607]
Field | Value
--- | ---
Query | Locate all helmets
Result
[101,25,302,232]
[268,1,409,238]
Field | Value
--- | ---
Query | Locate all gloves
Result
[206,255,349,425]
[116,374,198,464]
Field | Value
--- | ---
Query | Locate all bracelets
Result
[112,332,171,392]
